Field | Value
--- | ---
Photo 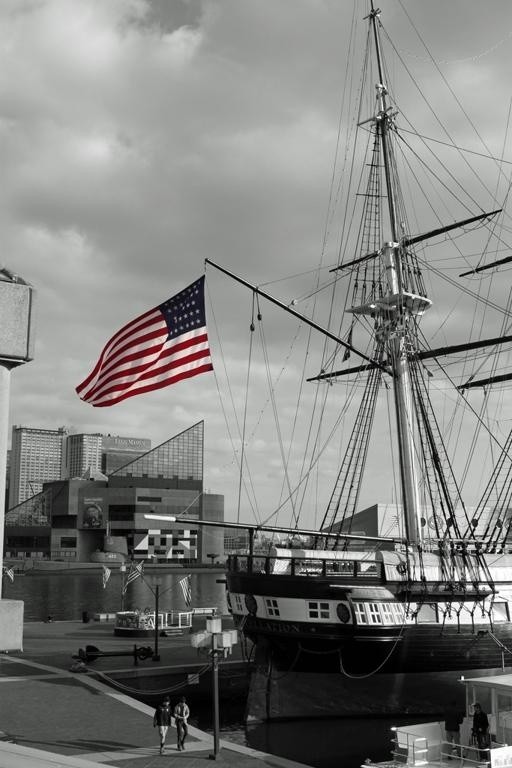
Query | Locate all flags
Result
[100,564,113,591]
[76,274,214,409]
[118,559,146,597]
[7,566,16,585]
[178,573,192,607]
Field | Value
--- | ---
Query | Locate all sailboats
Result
[175,2,512,746]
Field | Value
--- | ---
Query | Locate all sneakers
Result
[177,741,185,751]
[161,746,165,753]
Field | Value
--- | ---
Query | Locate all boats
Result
[349,670,512,768]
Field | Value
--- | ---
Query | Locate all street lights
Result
[149,573,163,662]
[185,606,241,762]
[119,559,127,613]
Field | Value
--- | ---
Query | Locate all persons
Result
[152,696,173,756]
[470,702,490,763]
[444,699,466,760]
[173,697,191,751]
